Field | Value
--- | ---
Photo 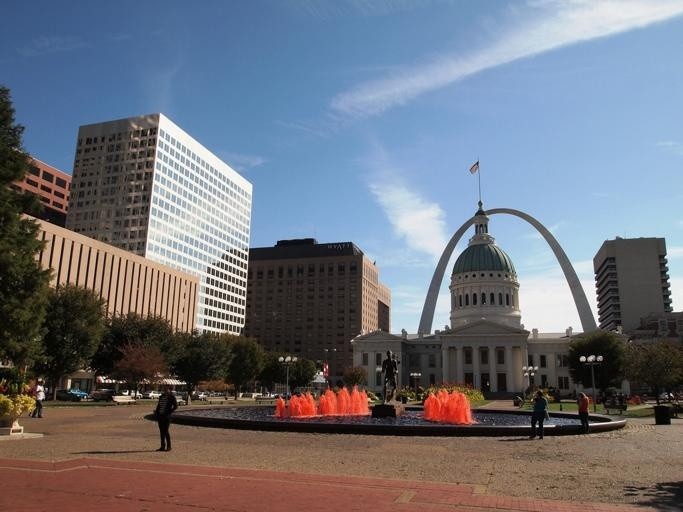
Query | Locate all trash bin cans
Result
[654,406,670,424]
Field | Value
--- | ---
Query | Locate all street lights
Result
[409,372,422,400]
[277,356,297,404]
[320,348,336,372]
[522,364,538,399]
[580,354,604,413]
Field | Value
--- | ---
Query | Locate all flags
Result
[468,160,479,176]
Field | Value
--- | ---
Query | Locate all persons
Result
[529,390,548,441]
[575,390,592,435]
[153,385,176,453]
[0,376,48,419]
[380,349,398,404]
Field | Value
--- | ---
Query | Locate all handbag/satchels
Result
[544,409,550,421]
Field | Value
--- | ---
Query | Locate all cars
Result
[54,386,224,402]
[658,392,674,400]
